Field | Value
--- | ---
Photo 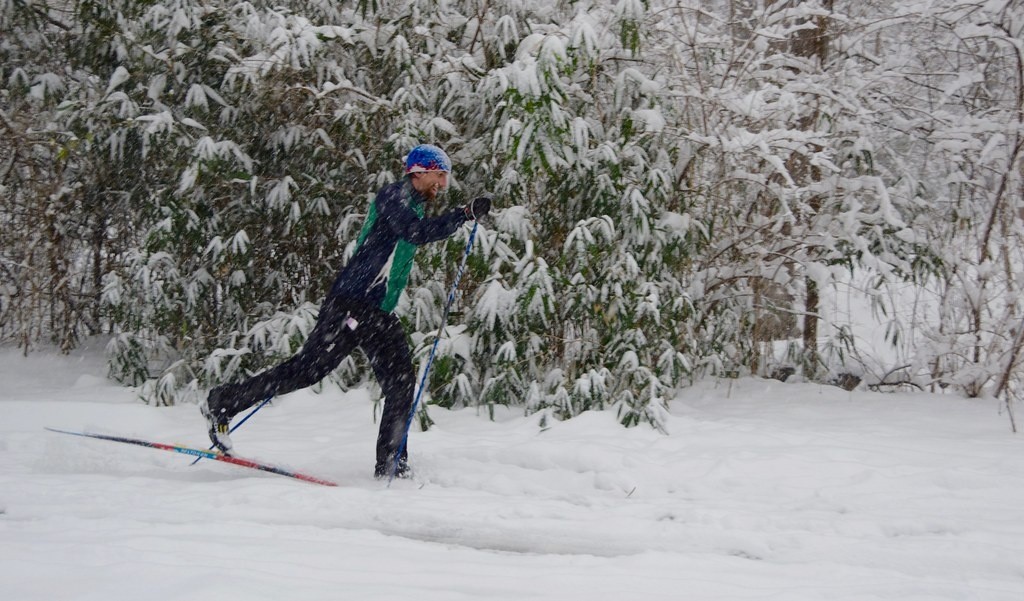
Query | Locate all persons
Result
[200,143,490,480]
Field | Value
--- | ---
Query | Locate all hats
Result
[405,144,451,175]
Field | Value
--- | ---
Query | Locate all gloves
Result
[459,197,491,222]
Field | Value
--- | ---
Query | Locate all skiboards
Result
[40,424,429,489]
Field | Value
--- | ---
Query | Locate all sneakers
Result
[200,399,233,452]
[374,462,414,480]
